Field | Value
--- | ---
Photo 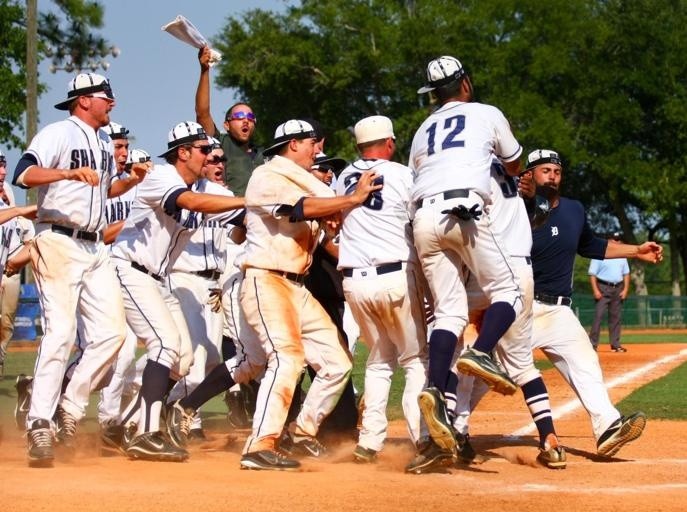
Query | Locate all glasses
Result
[227,111,257,122]
[313,166,334,172]
[88,87,115,101]
[209,154,228,164]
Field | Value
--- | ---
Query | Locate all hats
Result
[417,55,464,93]
[207,133,222,149]
[611,231,623,240]
[520,149,563,177]
[54,73,115,109]
[101,119,130,139]
[314,150,347,167]
[162,119,201,160]
[354,116,397,144]
[126,148,149,164]
[0,148,9,164]
[261,120,316,154]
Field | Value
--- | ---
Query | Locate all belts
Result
[132,260,163,283]
[275,270,305,287]
[598,280,620,288]
[534,293,573,306]
[414,192,485,209]
[50,223,98,244]
[198,268,223,282]
[339,262,405,276]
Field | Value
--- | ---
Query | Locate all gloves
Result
[441,199,479,233]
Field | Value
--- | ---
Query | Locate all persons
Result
[194,45,274,198]
[586,229,630,356]
[407,55,524,451]
[110,122,246,462]
[16,122,141,459]
[0,180,35,364]
[160,148,352,450]
[444,149,665,460]
[336,116,445,466]
[0,154,38,311]
[160,133,244,447]
[404,150,567,472]
[310,121,327,158]
[126,147,153,176]
[237,119,353,471]
[11,73,153,466]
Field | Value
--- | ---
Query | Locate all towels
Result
[160,10,222,67]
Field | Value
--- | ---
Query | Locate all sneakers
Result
[535,447,566,468]
[415,388,455,452]
[121,422,136,455]
[240,451,300,472]
[14,372,33,422]
[455,431,488,465]
[457,353,517,396]
[98,429,130,457]
[186,414,205,441]
[352,445,377,463]
[406,442,452,472]
[25,414,51,465]
[55,407,77,457]
[223,390,250,430]
[128,432,188,462]
[279,429,328,457]
[161,395,186,450]
[597,411,646,458]
[609,345,625,354]
